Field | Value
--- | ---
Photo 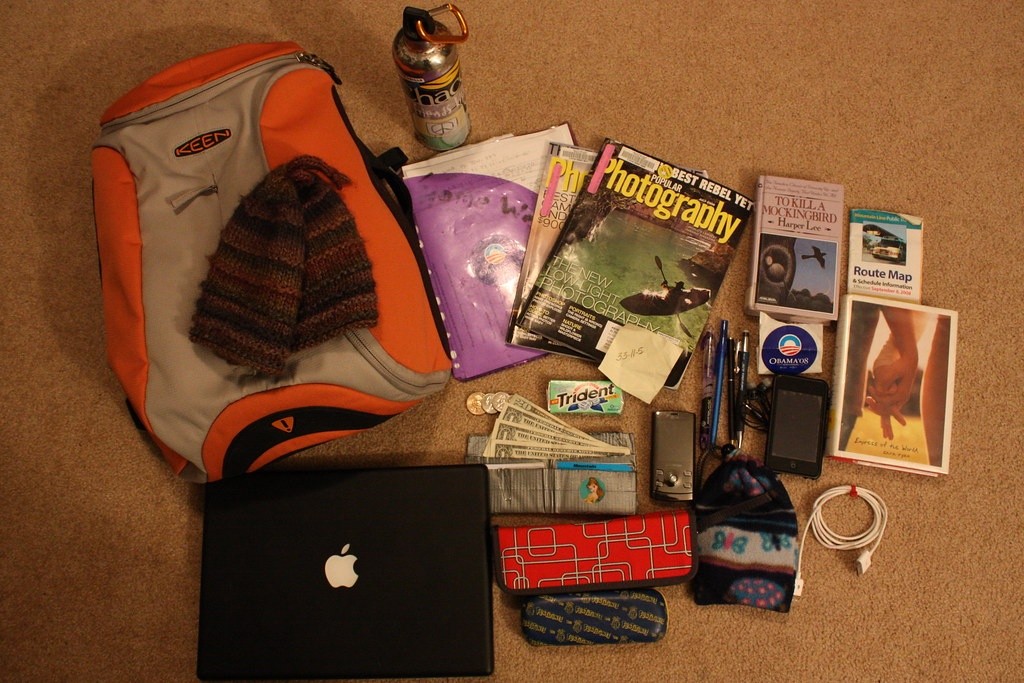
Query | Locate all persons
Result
[841,302,944,466]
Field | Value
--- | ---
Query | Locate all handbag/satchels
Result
[692,443,798,613]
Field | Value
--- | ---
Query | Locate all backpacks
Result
[90,41,453,484]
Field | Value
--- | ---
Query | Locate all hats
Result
[189,155,379,375]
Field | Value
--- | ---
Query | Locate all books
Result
[744,175,846,322]
[847,207,925,305]
[827,293,959,480]
[496,136,758,398]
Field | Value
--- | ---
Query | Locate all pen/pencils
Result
[710,320,750,449]
[700,331,714,450]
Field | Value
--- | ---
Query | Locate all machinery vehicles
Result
[871,235,906,263]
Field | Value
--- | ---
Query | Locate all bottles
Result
[391,4,473,152]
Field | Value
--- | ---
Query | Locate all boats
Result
[616,285,712,317]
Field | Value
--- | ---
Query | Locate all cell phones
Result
[766,375,828,479]
[650,410,696,501]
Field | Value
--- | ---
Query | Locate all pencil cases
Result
[493,507,699,595]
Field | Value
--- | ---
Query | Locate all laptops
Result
[197,463,495,683]
